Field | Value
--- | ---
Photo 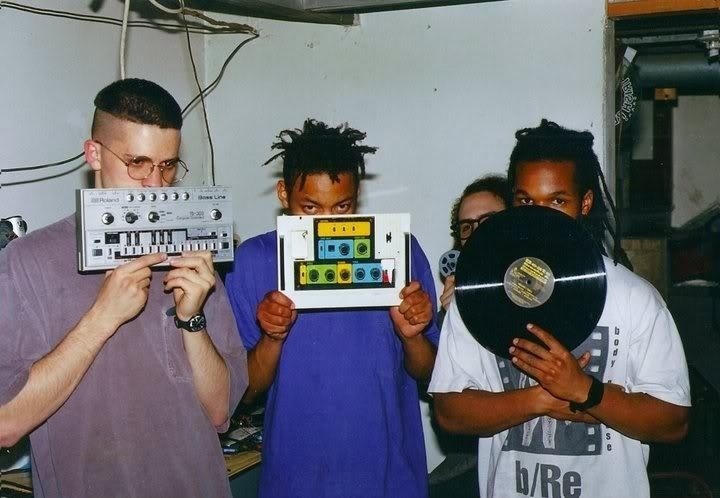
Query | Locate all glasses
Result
[455,212,492,240]
[94,139,188,184]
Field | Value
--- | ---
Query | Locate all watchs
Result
[171,313,208,333]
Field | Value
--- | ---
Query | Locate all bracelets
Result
[568,376,604,415]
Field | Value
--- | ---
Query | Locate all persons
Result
[426,173,519,329]
[426,116,696,497]
[0,76,248,497]
[221,119,440,497]
